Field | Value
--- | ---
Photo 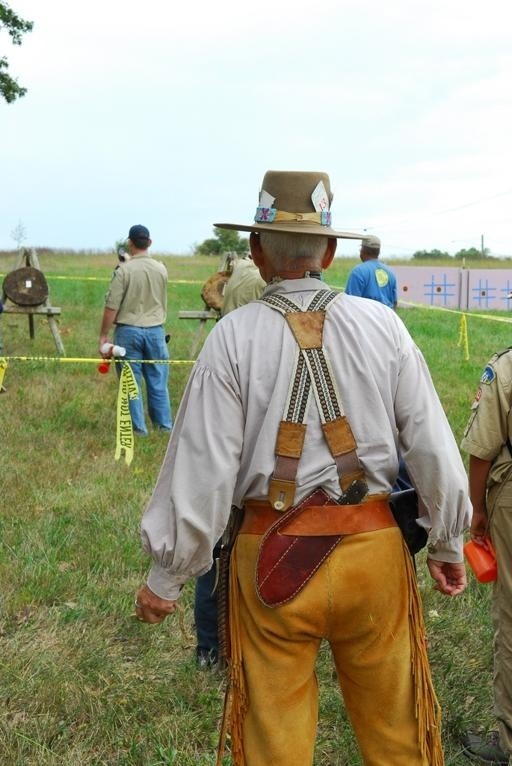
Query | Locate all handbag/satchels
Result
[189,545,232,670]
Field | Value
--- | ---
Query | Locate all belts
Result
[236,498,401,538]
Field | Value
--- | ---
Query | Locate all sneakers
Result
[459,730,509,765]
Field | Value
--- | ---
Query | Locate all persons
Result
[192,536,229,671]
[100,223,173,436]
[344,237,398,312]
[134,166,478,766]
[220,255,270,323]
[458,343,511,763]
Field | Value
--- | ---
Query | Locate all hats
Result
[360,234,382,250]
[212,167,371,247]
[126,223,152,240]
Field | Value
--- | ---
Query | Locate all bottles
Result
[98,357,111,374]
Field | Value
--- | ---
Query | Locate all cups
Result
[462,536,497,583]
[101,343,127,357]
